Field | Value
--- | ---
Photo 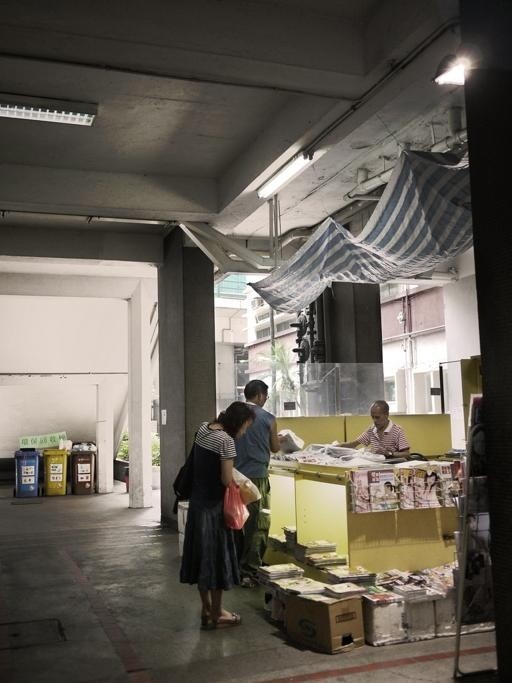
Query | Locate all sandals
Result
[201,612,244,629]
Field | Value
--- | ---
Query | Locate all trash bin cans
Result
[13,442,96,498]
[114,460,129,482]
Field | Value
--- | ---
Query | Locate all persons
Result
[466,513,494,619]
[179,400,257,629]
[330,399,411,458]
[227,379,289,588]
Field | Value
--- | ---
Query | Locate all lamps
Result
[0,92,99,126]
[257,152,313,201]
[432,53,466,87]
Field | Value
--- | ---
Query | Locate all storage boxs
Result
[364,596,436,647]
[179,533,185,557]
[436,597,495,637]
[284,594,364,655]
[177,501,189,534]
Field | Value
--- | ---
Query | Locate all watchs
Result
[387,450,393,457]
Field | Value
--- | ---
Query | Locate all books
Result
[347,458,468,513]
[255,511,457,647]
[271,442,387,467]
[277,429,304,442]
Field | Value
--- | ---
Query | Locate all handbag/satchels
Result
[172,457,191,500]
[225,479,250,530]
[232,468,262,505]
